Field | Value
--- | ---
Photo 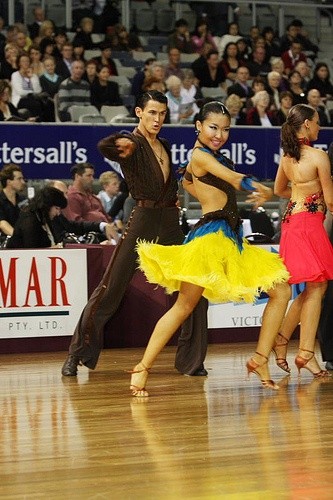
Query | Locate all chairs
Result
[54,32,254,125]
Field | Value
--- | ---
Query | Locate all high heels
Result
[294,349,330,377]
[123,361,152,397]
[246,352,278,391]
[272,332,292,372]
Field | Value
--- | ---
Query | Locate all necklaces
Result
[3,188,14,201]
[153,142,164,166]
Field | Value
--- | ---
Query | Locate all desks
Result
[0,241,306,354]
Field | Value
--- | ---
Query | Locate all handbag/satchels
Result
[62,231,100,246]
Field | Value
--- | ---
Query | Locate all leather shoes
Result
[61,358,79,376]
[194,368,208,376]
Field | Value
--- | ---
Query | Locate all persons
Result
[0,164,136,249]
[0,0,333,127]
[270,104,333,377]
[314,140,333,371]
[61,91,209,377]
[128,101,292,396]
